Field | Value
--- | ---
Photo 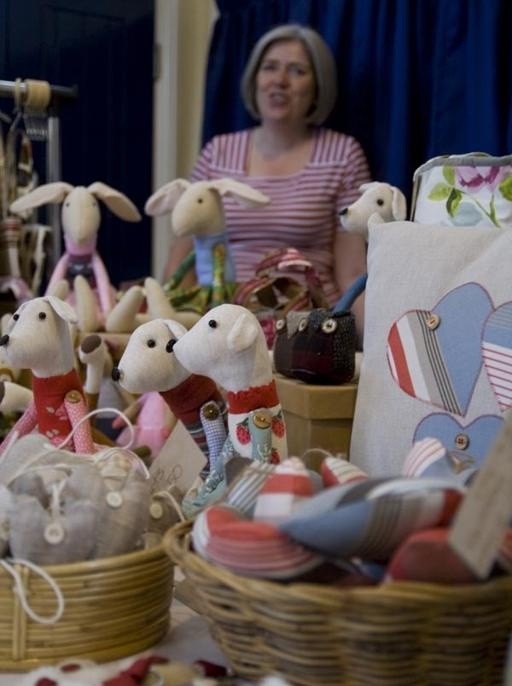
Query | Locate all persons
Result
[158,23,371,353]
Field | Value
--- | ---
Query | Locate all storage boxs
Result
[270,369,357,472]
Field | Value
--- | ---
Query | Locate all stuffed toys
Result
[0,178,511,584]
